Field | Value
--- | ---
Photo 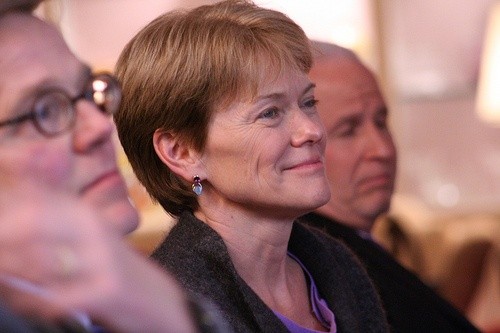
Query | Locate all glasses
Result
[0,71,122,139]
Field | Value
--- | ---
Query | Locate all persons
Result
[1,1,230,332]
[299,40,483,333]
[108,2,392,333]
